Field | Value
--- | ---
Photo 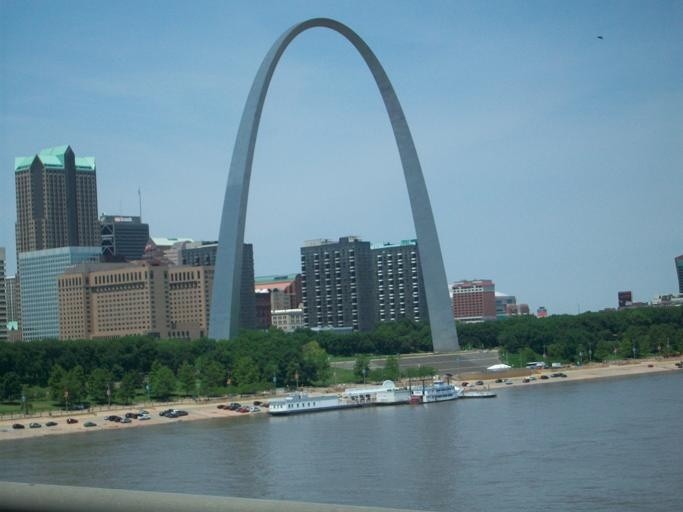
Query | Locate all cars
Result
[83,421,96,427]
[495,378,514,384]
[217,401,261,413]
[67,417,78,424]
[158,408,188,418]
[12,423,25,429]
[46,421,58,426]
[29,422,42,428]
[103,410,151,423]
[461,380,484,387]
[522,372,567,383]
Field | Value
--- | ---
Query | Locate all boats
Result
[457,387,497,397]
[267,392,364,416]
[411,380,458,404]
